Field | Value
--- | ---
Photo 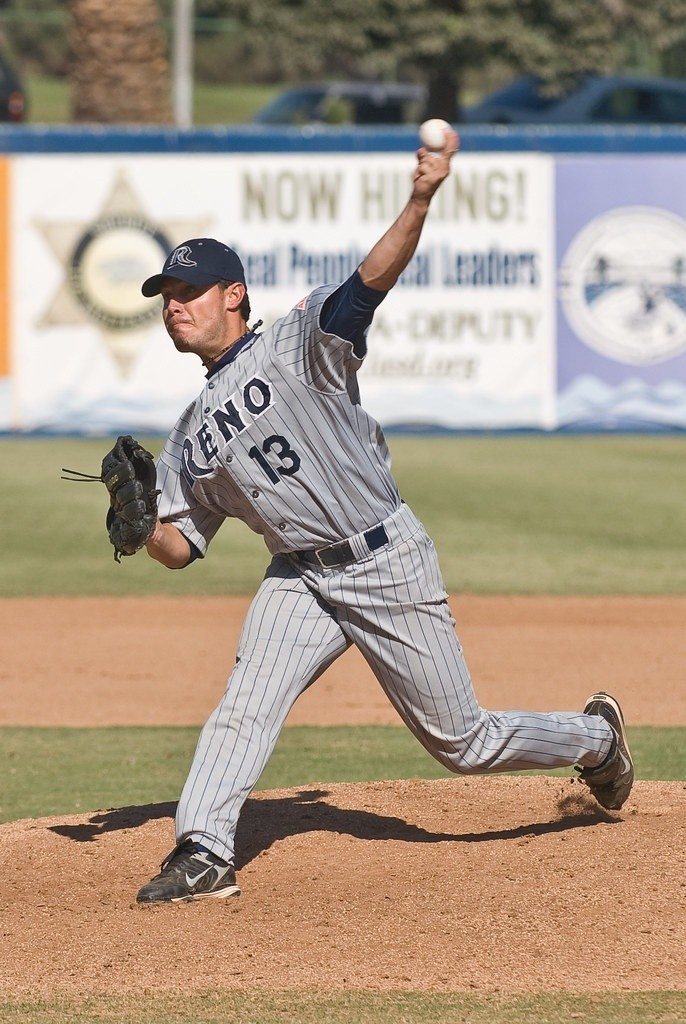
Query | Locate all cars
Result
[466,70,685,127]
[248,81,428,124]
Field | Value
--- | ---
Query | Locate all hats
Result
[141,238,246,297]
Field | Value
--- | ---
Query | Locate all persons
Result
[136,126,634,905]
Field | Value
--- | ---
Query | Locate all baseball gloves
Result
[101,437,164,558]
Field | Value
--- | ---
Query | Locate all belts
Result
[293,499,405,568]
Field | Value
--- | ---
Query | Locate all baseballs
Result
[417,118,454,151]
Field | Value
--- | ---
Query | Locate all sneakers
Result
[573,690,635,811]
[136,837,241,904]
